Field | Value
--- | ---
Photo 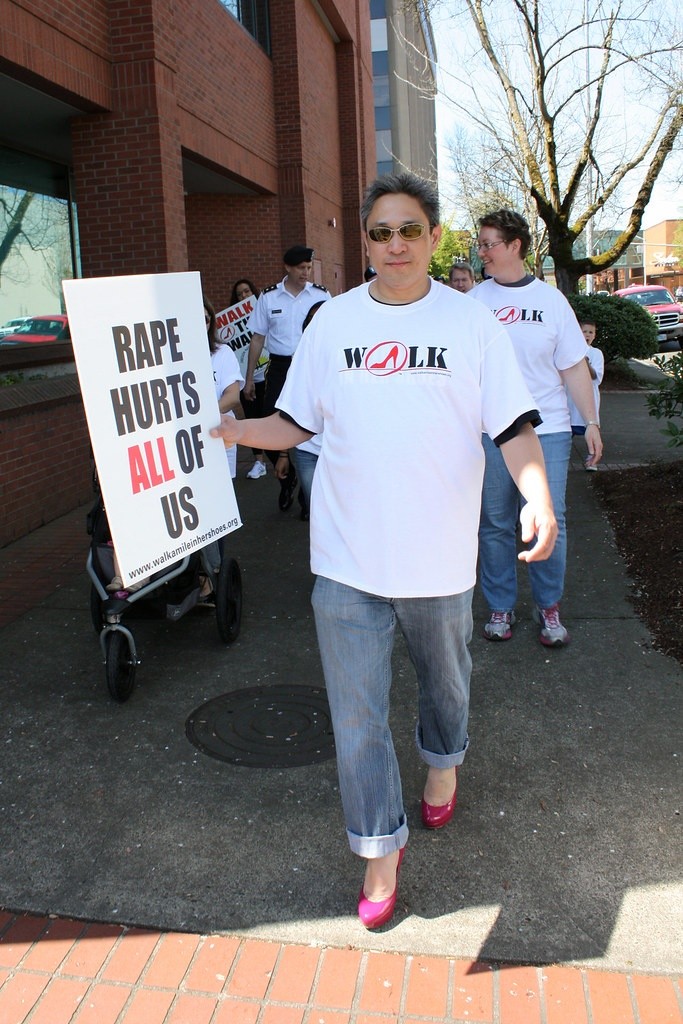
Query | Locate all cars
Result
[0,315,70,345]
[0,319,25,339]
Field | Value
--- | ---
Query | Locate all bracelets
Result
[586,420,600,429]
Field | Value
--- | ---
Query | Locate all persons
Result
[200,172,604,929]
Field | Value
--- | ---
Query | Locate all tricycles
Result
[85,441,243,703]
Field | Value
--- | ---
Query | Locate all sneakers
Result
[536,603,570,648]
[483,611,518,642]
[246,461,267,481]
[582,455,601,471]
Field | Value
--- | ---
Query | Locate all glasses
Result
[478,240,509,251]
[365,223,435,244]
[205,316,211,324]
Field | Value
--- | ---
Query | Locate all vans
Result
[597,291,610,297]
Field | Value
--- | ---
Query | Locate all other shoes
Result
[123,577,150,593]
[105,577,122,590]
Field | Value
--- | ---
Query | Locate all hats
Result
[283,245,315,267]
[364,267,377,279]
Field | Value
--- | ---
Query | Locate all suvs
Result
[613,285,683,349]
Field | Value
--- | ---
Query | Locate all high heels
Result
[356,847,407,932]
[420,769,457,832]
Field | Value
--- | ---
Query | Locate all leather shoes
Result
[278,473,298,512]
[298,485,310,521]
